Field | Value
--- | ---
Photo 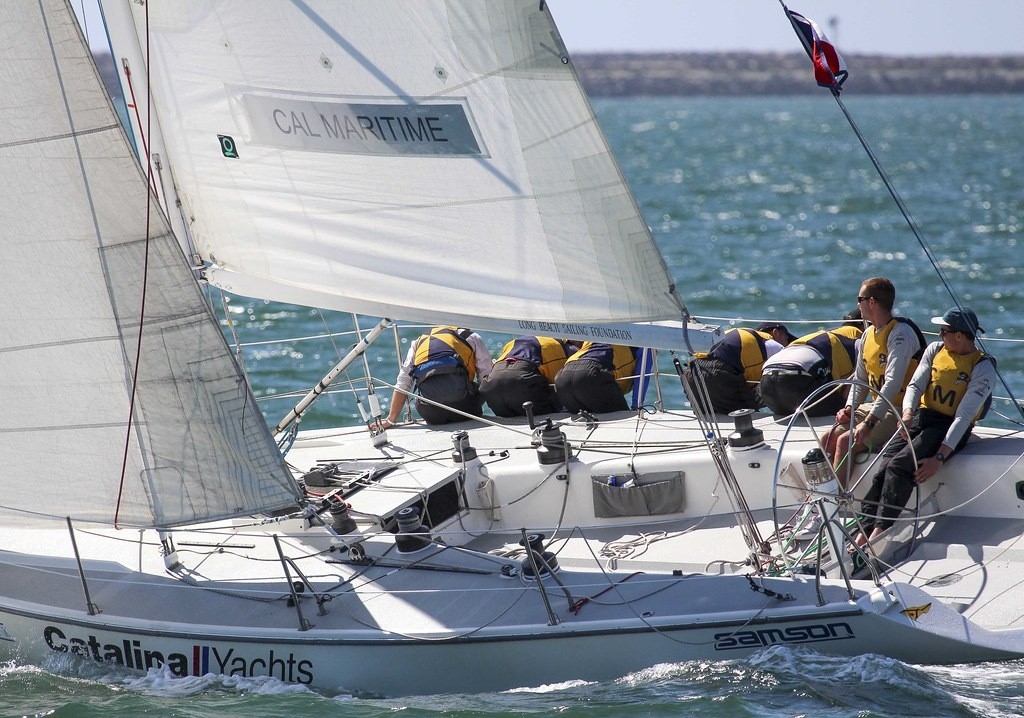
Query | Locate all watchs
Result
[864,419,875,430]
[936,451,946,464]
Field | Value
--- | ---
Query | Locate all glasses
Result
[941,328,963,335]
[858,297,879,304]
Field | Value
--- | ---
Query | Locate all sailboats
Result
[0,1,1024,700]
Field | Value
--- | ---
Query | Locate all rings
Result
[836,412,840,414]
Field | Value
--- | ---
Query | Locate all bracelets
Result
[385,417,396,426]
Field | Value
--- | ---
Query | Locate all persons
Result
[759,308,872,417]
[784,278,930,540]
[848,307,997,555]
[679,321,798,415]
[554,341,654,413]
[369,325,492,431]
[479,336,581,416]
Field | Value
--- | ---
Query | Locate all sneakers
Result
[785,511,825,541]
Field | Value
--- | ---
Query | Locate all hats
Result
[930,306,986,335]
[757,322,798,344]
[849,308,862,319]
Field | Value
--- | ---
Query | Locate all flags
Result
[783,5,849,97]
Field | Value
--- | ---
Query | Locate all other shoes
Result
[847,548,870,575]
